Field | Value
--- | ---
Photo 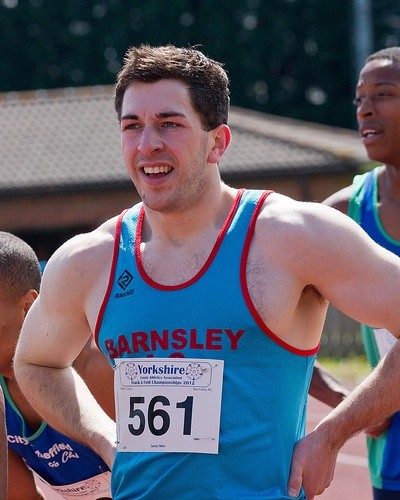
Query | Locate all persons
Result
[308,47,400,500]
[13,45,400,500]
[0,231,116,500]
